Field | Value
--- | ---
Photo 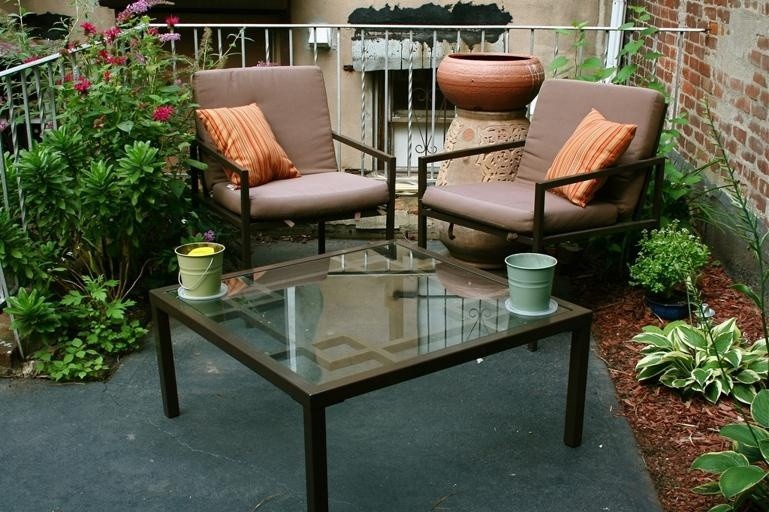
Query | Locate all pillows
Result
[194,102,301,189]
[544,108,637,208]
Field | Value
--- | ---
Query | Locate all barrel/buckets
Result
[174,242,227,297]
[503,254,558,312]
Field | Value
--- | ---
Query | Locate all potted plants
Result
[626,218,711,321]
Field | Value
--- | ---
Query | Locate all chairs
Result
[190,65,397,329]
[417,79,668,352]
